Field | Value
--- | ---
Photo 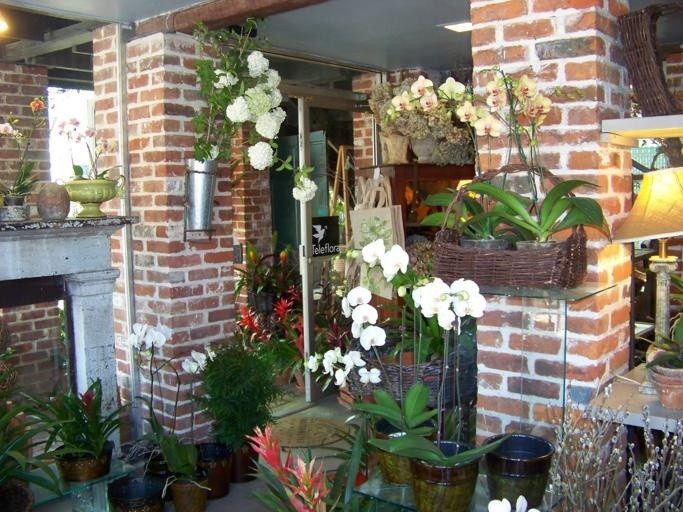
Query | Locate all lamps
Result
[610,163,681,371]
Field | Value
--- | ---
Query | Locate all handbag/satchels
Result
[349,172,409,303]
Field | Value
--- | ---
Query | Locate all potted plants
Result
[2,373,137,510]
[636,313,683,412]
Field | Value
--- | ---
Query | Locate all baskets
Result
[430,164,588,295]
[343,322,478,408]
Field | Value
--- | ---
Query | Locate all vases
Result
[483,434,552,512]
[4,194,25,207]
[411,439,478,512]
[108,441,232,512]
[66,176,127,218]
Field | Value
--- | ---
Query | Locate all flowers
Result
[58,117,126,184]
[546,378,683,512]
[245,425,346,512]
[0,97,45,196]
